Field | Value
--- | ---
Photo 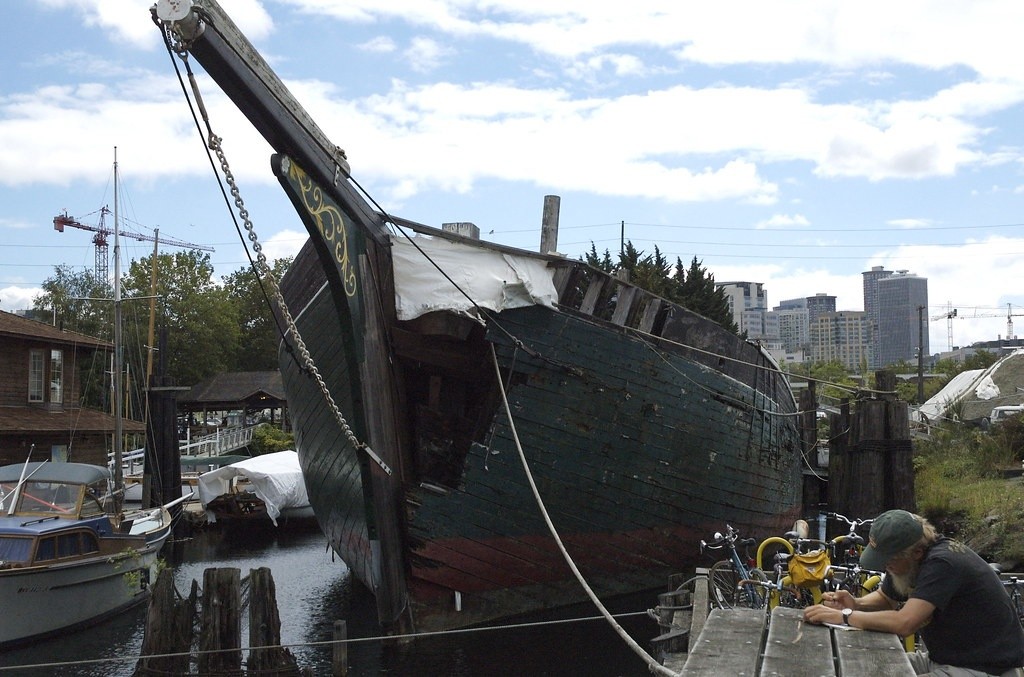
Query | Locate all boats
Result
[197,449,316,523]
[152,0,804,654]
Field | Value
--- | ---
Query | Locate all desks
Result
[679,605,916,677]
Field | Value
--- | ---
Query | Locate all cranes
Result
[53,204,217,295]
[932,298,957,352]
[955,301,1024,340]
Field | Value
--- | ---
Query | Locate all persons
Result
[805,510,1024,677]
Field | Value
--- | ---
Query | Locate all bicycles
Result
[698,510,1024,655]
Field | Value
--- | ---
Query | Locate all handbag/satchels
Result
[789,548,835,587]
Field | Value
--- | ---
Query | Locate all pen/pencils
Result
[833,583,840,604]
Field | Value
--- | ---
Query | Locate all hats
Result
[859,509,923,570]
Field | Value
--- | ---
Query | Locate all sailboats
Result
[1,146,195,652]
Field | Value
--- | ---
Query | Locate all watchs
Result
[842,608,853,627]
